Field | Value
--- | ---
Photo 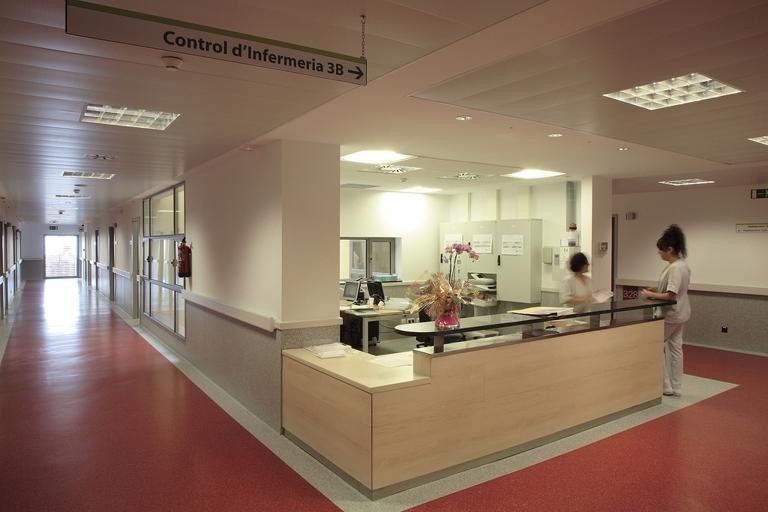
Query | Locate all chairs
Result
[416,308,461,348]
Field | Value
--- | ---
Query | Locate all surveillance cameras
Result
[81,224,85,226]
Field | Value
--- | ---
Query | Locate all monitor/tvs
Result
[342,281,361,301]
[366,281,385,304]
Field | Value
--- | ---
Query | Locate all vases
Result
[435,303,460,330]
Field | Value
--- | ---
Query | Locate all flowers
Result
[399,243,484,323]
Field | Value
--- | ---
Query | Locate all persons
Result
[559,252,600,306]
[640,224,691,398]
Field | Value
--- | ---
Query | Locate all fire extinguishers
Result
[177,237,192,277]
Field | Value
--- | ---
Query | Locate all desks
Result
[340,297,410,353]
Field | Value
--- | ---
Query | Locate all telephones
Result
[371,294,384,305]
